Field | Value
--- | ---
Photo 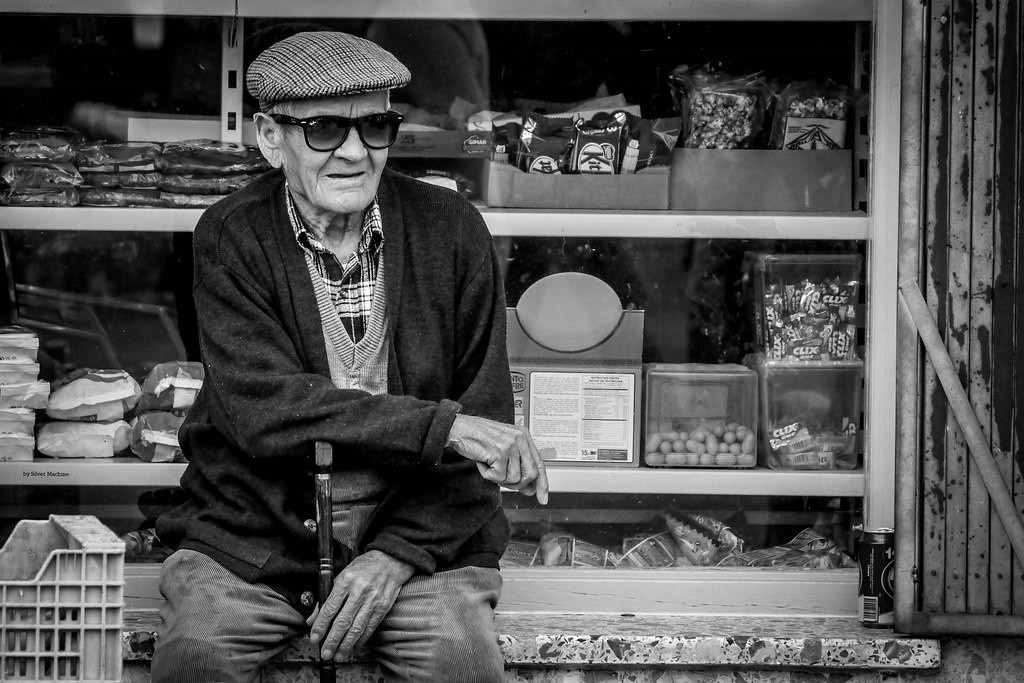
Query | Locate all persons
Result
[150,32,549,683]
[366,20,488,114]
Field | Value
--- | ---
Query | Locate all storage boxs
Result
[670,148,852,212]
[642,362,758,468]
[469,157,670,211]
[506,272,644,469]
[0,514,125,683]
[746,251,862,361]
[743,356,865,470]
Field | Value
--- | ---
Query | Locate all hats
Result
[247,33,412,109]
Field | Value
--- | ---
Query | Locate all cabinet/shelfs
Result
[0,0,899,547]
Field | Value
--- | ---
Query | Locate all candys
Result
[646,421,755,467]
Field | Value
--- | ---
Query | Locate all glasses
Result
[269,109,404,149]
